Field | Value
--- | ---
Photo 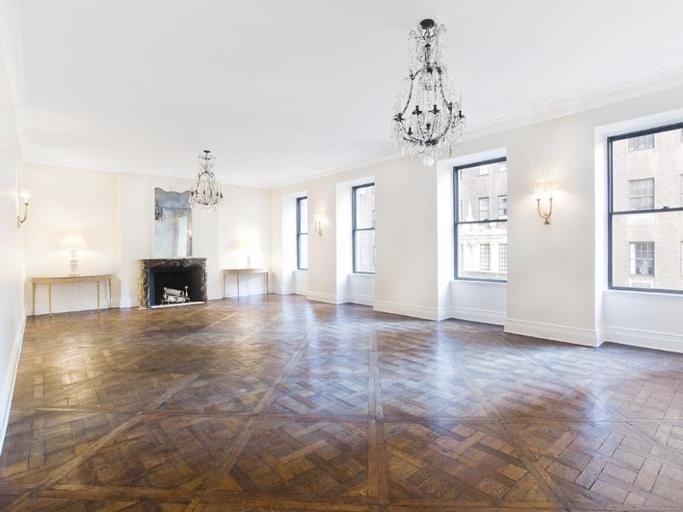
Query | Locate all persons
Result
[640,261,649,274]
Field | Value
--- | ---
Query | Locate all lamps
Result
[17,186,32,227]
[390,18,467,166]
[189,150,224,209]
[534,182,556,224]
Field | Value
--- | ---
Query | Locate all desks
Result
[32,274,113,317]
[222,267,268,302]
